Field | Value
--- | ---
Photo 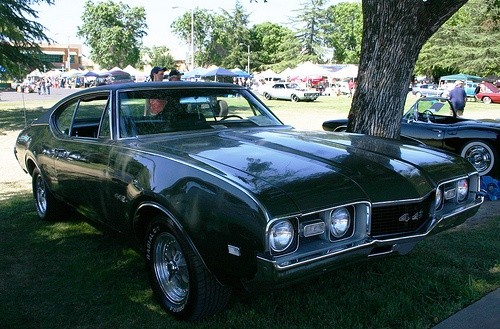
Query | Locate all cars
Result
[13,80,485,325]
[412,79,500,104]
[254,81,321,102]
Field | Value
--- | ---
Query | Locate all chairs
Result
[171,113,207,130]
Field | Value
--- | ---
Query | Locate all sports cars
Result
[322,97,499,176]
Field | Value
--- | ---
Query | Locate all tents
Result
[26,64,143,80]
[439,75,483,82]
[183,64,360,95]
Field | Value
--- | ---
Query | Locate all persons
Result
[51,78,110,88]
[37,77,51,95]
[144,67,167,116]
[167,70,181,104]
[446,80,467,116]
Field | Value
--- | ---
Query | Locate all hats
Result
[151,67,167,75]
[455,80,463,86]
[169,69,184,77]
